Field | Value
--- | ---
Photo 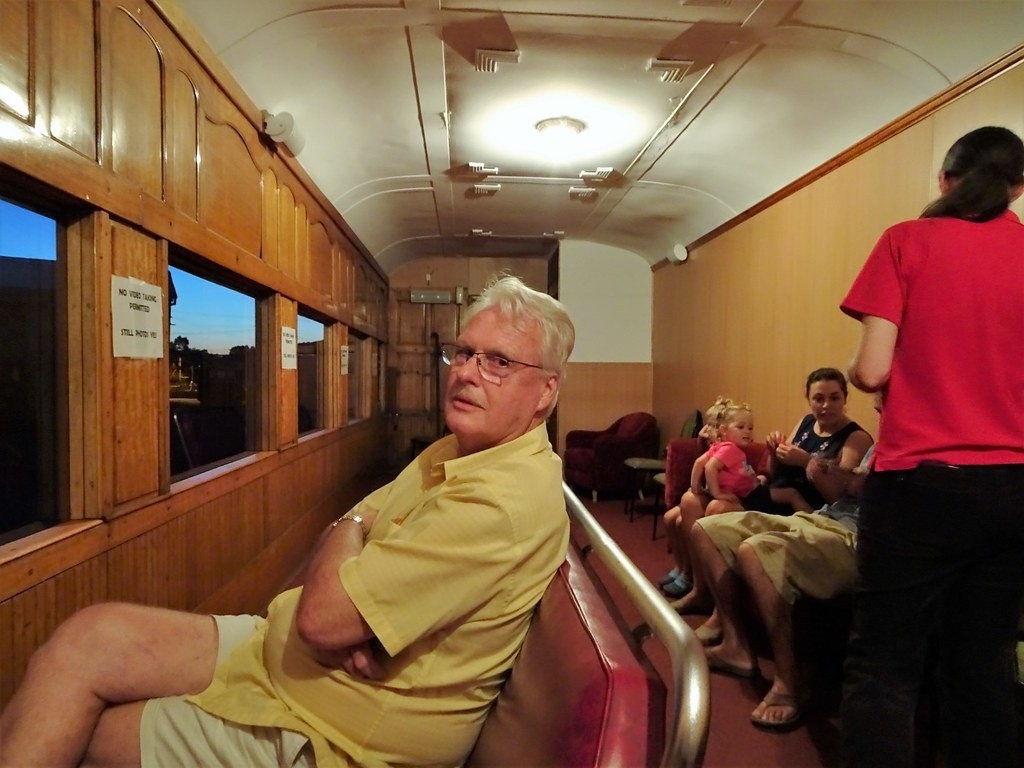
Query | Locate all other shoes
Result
[670,599,709,614]
[694,625,723,648]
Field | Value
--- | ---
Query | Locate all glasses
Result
[442,342,543,385]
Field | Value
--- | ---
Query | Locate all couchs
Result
[666,437,773,563]
[563,411,654,497]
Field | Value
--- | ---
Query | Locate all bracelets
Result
[333,514,367,538]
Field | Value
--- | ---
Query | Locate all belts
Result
[897,465,1018,489]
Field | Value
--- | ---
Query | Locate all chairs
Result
[624,409,702,521]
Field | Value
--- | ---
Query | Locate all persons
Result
[701,381,891,726]
[690,404,813,514]
[670,367,875,644]
[840,127,1024,768]
[0,279,577,768]
[657,398,774,599]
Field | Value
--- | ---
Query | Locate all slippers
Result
[750,691,809,731]
[703,647,758,679]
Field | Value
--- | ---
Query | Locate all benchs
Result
[468,480,711,768]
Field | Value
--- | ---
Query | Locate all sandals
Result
[658,569,693,597]
